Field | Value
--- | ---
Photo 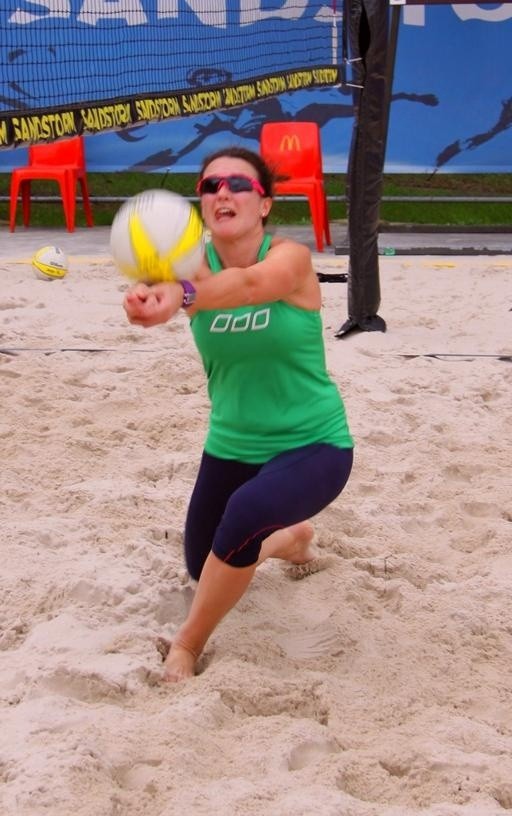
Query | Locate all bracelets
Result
[178,279,198,310]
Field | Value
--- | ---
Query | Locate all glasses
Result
[196,171,266,197]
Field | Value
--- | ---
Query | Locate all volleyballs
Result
[31,246,68,281]
[109,188,204,283]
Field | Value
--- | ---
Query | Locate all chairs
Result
[258,121,332,253]
[9,137,95,232]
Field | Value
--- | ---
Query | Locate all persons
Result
[123,147,355,682]
